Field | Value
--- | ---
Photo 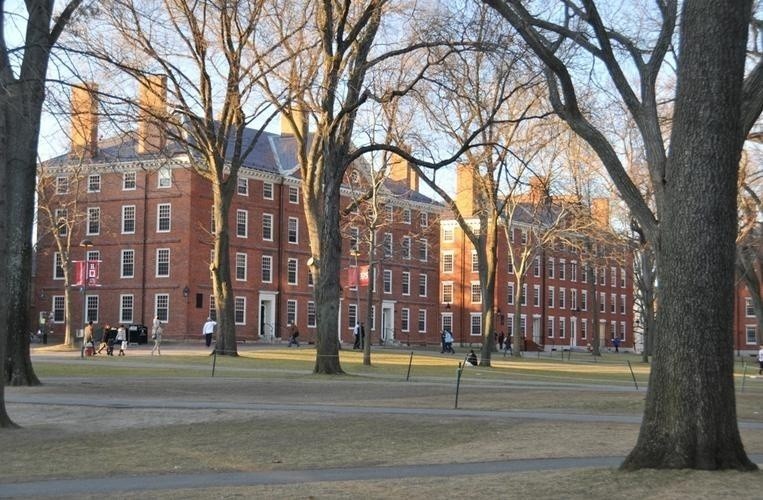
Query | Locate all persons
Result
[202,317,217,347]
[115,324,125,356]
[286,320,300,347]
[497,331,504,350]
[352,321,364,349]
[503,331,512,357]
[757,345,763,375]
[352,321,361,345]
[78,321,96,359]
[439,328,455,354]
[96,324,109,355]
[105,327,118,356]
[150,320,162,356]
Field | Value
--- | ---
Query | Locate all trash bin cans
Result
[128,324,148,345]
[83,341,94,357]
